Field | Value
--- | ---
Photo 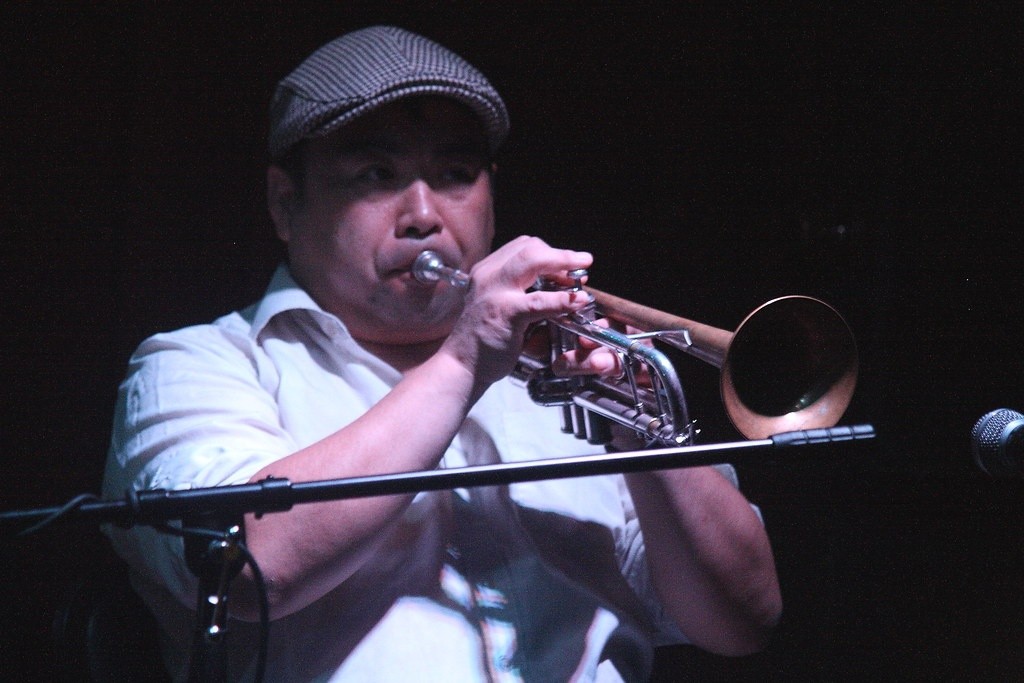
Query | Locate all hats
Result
[271,25,510,167]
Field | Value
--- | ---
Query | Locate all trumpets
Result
[403,244,862,450]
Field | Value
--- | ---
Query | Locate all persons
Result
[104,24,782,683]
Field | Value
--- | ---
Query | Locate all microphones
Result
[970,407,1024,480]
